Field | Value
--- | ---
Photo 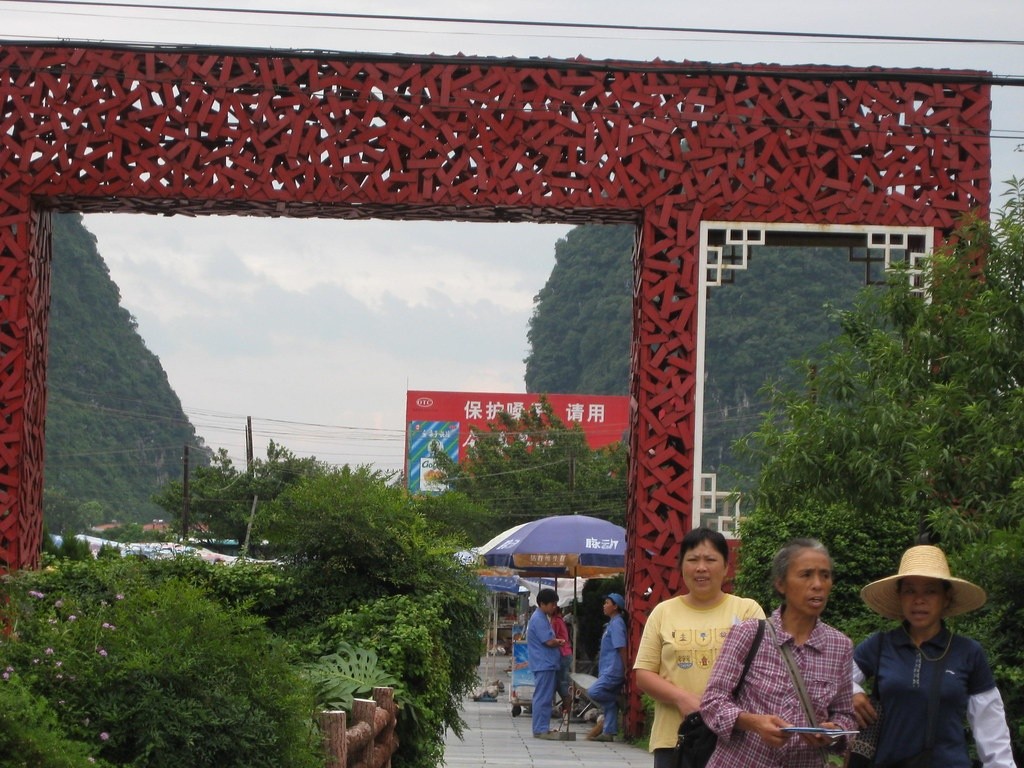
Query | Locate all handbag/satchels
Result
[846,630,885,768]
[674,710,718,768]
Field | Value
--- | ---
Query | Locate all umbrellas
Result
[435,545,560,688]
[475,515,628,717]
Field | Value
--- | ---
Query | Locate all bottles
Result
[519,612,527,623]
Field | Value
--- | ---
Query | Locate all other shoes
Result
[590,734,613,742]
[540,733,561,739]
[533,732,540,737]
[620,695,626,715]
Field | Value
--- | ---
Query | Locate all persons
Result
[525,588,566,740]
[546,606,572,711]
[587,592,631,743]
[632,525,767,768]
[698,536,860,768]
[844,543,1023,768]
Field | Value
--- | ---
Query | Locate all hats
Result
[608,593,625,609]
[860,545,987,622]
